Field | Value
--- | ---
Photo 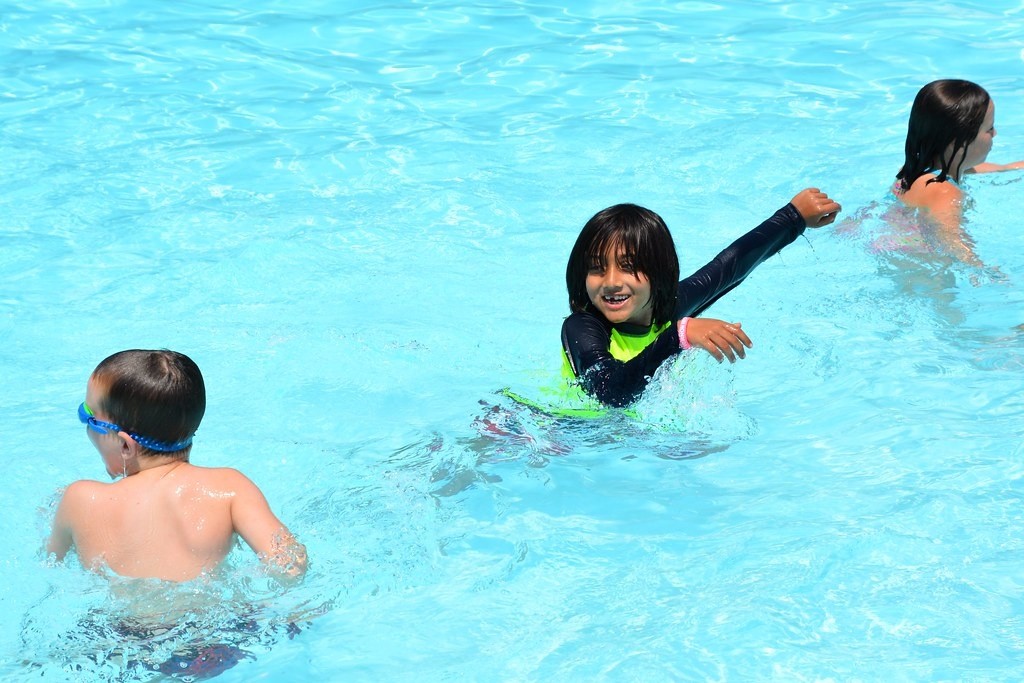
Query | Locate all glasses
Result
[78,401,193,453]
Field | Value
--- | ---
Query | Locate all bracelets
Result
[677,316,692,351]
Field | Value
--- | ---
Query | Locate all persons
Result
[893,78,1024,229]
[45,350,308,588]
[557,187,842,409]
